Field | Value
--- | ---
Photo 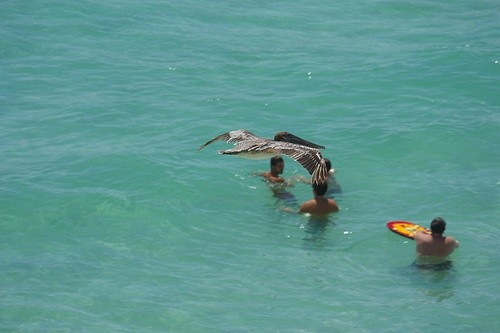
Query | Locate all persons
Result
[254,156,342,216]
[408,217,462,273]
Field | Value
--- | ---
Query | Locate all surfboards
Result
[386,220,433,240]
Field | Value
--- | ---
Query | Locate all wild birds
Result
[197,129,328,186]
